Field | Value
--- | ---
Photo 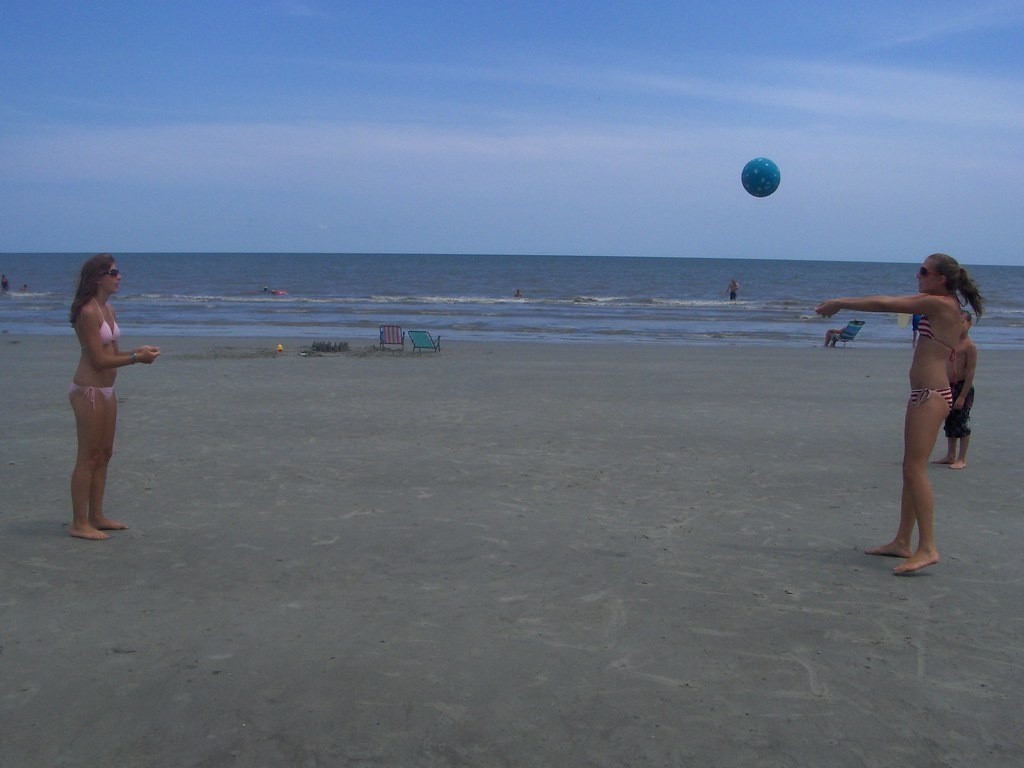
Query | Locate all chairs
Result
[409,330,440,353]
[830,320,865,348]
[380,326,404,351]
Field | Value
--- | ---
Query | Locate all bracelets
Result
[130,352,136,364]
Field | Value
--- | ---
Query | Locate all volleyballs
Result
[740,157,781,198]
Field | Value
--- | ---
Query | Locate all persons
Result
[931,310,978,468]
[814,252,984,574]
[912,314,923,348]
[20,283,27,291]
[1,275,7,291]
[824,319,858,347]
[726,280,739,301]
[514,289,522,297]
[264,287,284,295]
[67,253,160,540]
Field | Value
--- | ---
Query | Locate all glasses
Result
[920,267,940,279]
[104,269,119,276]
